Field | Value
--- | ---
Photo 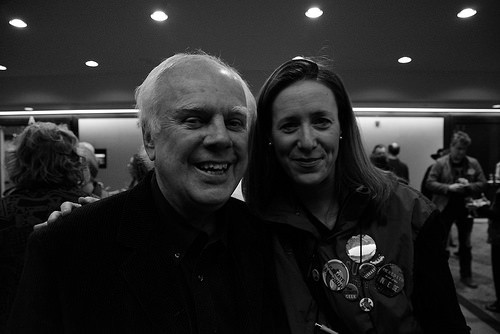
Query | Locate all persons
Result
[0,120,114,334]
[369,130,500,313]
[6,52,292,334]
[32,57,473,334]
